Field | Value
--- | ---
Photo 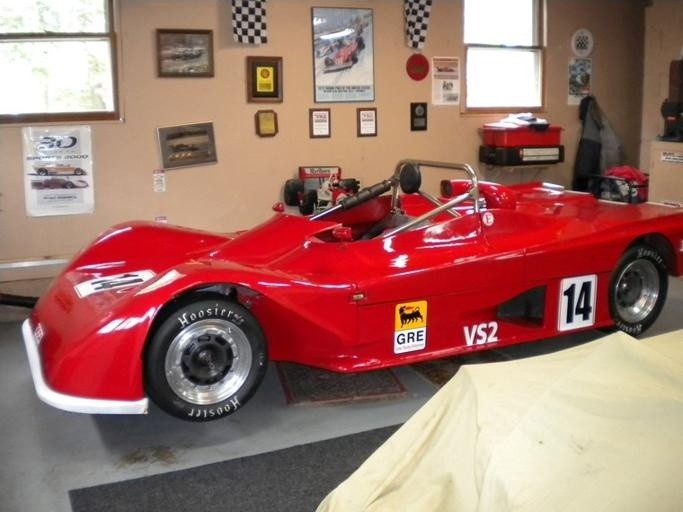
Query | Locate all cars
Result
[171,47,203,63]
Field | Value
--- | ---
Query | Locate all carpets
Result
[276,362,407,409]
[68,423,405,511]
[412,349,509,390]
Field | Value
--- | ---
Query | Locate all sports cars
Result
[437,66,457,73]
[168,143,200,151]
[32,162,87,177]
[20,160,682,426]
[314,36,366,73]
[31,178,89,188]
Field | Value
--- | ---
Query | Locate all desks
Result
[496,164,560,186]
[646,141,683,206]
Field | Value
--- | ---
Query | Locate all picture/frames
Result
[256,109,277,137]
[357,108,377,136]
[156,122,217,168]
[156,27,214,77]
[310,108,331,138]
[246,56,282,102]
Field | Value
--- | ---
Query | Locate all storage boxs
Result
[479,146,565,166]
[483,125,563,148]
